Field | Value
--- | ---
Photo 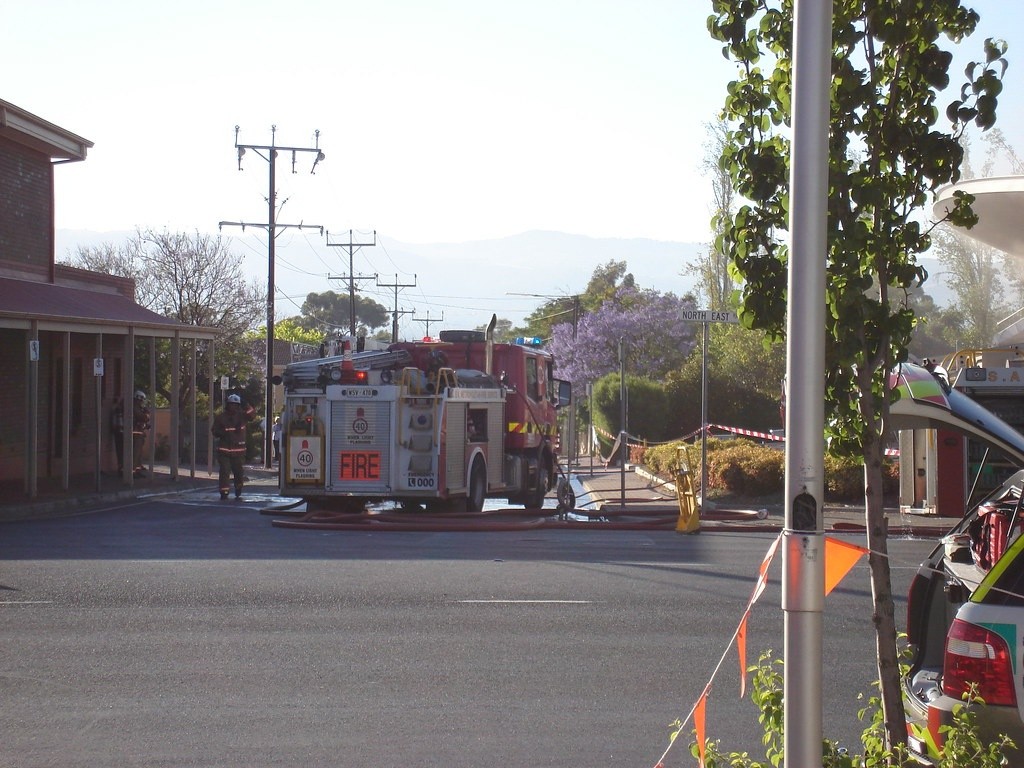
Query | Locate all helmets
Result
[134,391,146,401]
[227,394,241,403]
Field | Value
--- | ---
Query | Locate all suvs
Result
[892,359,1023,768]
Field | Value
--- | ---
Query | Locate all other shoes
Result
[136,471,147,479]
[133,472,141,479]
[219,489,229,496]
[235,485,242,497]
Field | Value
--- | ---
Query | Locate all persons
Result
[113,390,151,479]
[210,394,256,500]
[273,416,281,460]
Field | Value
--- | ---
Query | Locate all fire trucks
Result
[273,313,574,515]
